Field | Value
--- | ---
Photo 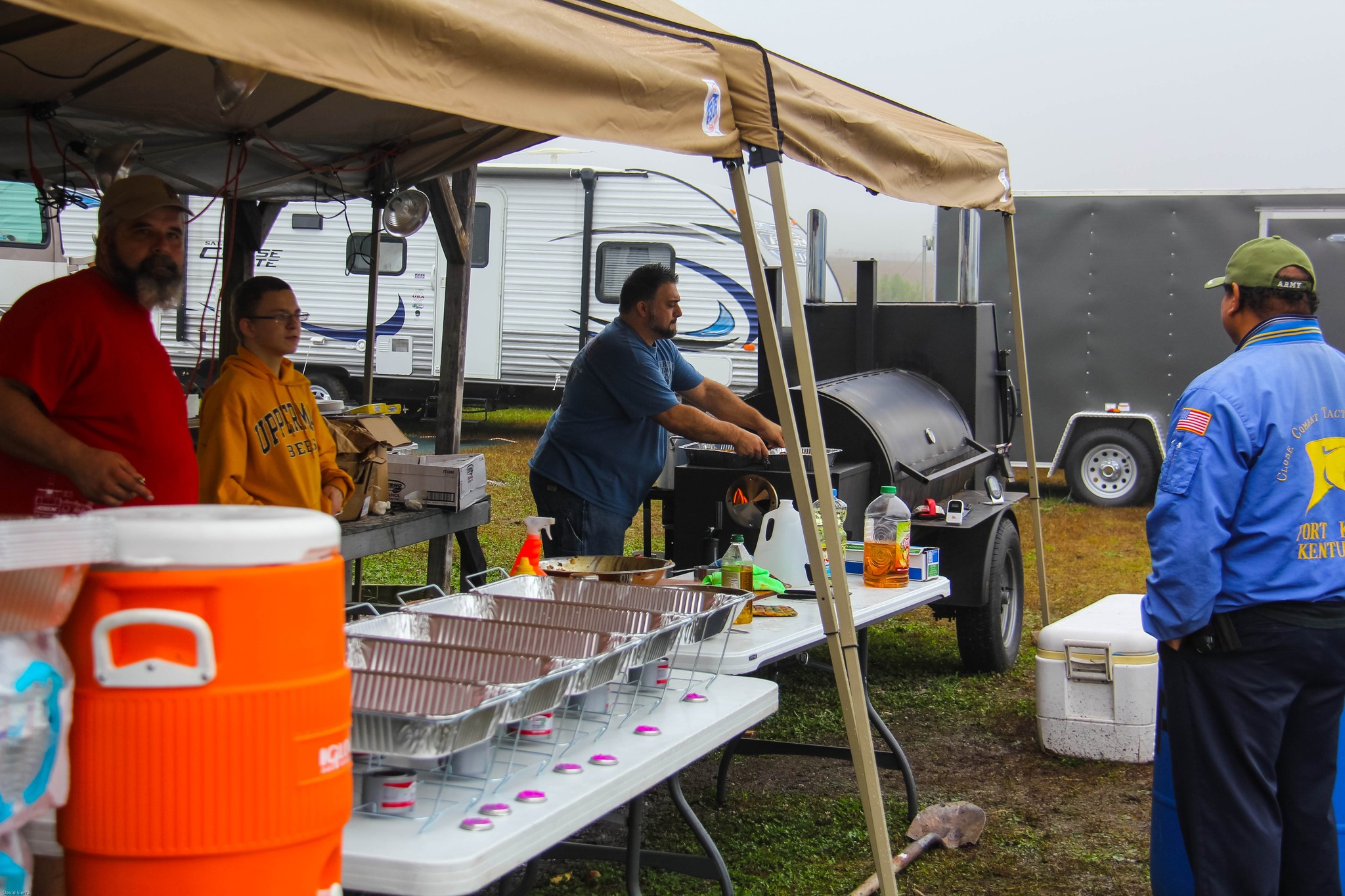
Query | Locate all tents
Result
[0,0,1049,896]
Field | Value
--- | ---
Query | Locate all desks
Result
[339,665,782,896]
[501,571,949,896]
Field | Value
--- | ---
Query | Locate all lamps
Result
[70,136,145,194]
[209,58,268,116]
[382,189,429,236]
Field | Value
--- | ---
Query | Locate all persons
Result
[1142,234,1345,896]
[527,262,784,558]
[196,276,355,517]
[0,176,200,520]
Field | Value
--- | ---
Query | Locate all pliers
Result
[777,589,852,600]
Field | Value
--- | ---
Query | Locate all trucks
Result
[937,198,1344,507]
[0,155,844,421]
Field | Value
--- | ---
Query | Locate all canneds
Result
[506,712,554,742]
[360,770,419,816]
[565,685,611,716]
[628,657,669,689]
[437,740,490,775]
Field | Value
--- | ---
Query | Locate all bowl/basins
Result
[1,515,120,634]
[538,554,674,586]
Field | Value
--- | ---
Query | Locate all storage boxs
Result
[338,413,487,521]
[1034,594,1158,765]
[845,540,940,582]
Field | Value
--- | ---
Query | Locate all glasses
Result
[244,311,311,323]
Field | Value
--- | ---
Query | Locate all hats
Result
[98,174,195,231]
[1204,235,1316,294]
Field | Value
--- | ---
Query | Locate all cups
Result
[438,738,493,777]
[184,394,199,419]
[629,656,669,688]
[353,751,385,761]
[564,683,611,714]
[506,709,555,739]
[569,572,599,581]
[359,768,418,815]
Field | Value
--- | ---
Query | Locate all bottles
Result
[753,499,810,588]
[721,534,754,624]
[512,557,536,576]
[863,486,911,588]
[812,489,848,584]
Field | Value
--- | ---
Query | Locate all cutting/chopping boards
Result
[654,577,778,602]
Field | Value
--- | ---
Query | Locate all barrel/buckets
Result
[632,551,665,559]
[53,506,353,895]
[657,429,692,491]
